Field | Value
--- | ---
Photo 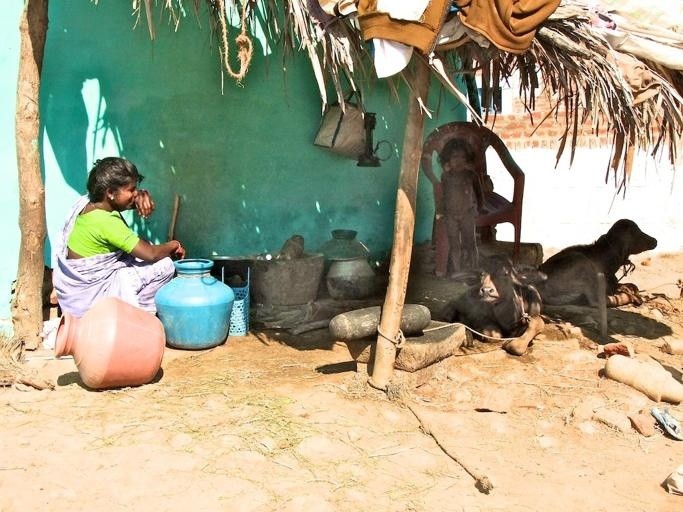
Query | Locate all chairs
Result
[420,121,525,278]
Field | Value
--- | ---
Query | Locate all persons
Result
[53,157,186,316]
[439,138,489,278]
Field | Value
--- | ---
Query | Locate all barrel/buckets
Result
[53,297,165,391]
[155,259,234,350]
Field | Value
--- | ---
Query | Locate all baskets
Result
[221,266,251,337]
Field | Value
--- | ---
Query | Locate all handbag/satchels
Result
[313,89,370,160]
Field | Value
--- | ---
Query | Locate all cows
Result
[529,219,657,308]
[430,253,548,357]
